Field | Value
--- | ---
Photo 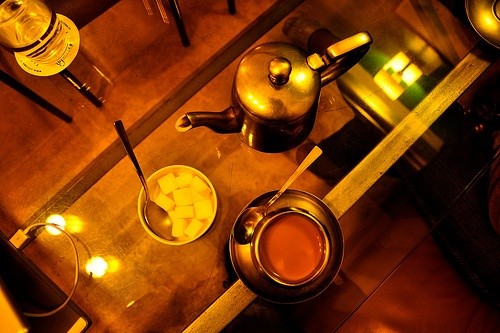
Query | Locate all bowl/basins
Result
[137,164,217,245]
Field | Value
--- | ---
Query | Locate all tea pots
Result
[174,31,372,152]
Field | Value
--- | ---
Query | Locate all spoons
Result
[114,120,175,241]
[234,146,322,245]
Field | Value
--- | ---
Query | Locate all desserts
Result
[153,173,213,239]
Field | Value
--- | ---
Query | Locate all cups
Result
[0,0,69,63]
[250,208,330,287]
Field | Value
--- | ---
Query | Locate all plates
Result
[228,189,344,304]
[14,12,81,77]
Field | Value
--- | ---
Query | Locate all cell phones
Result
[0,231,92,333]
